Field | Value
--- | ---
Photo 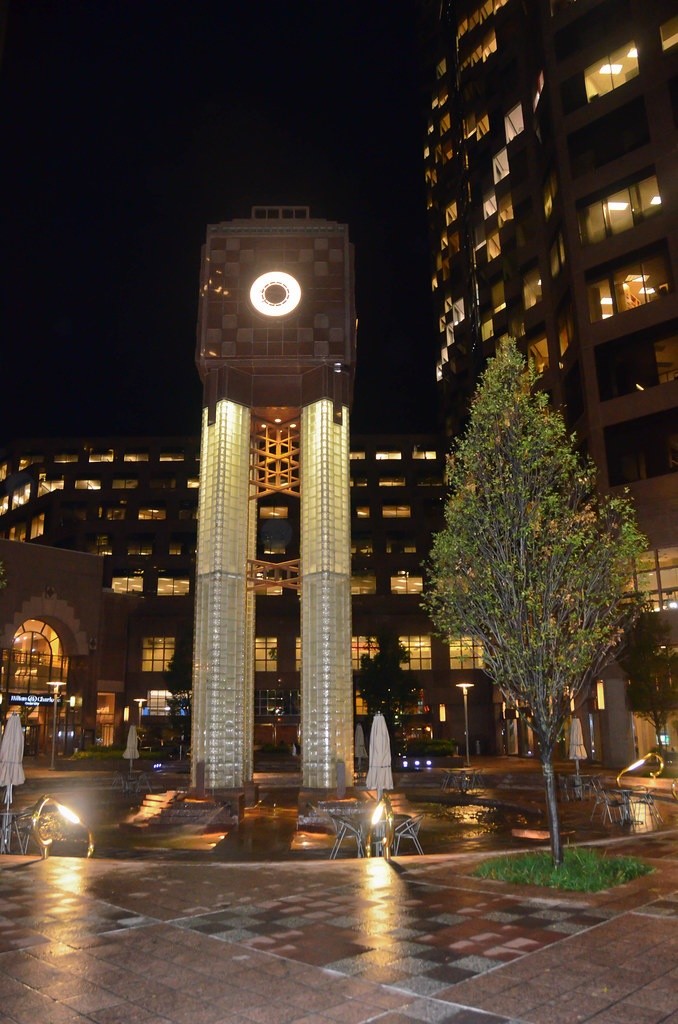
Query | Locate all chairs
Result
[3,805,53,854]
[111,769,153,793]
[441,768,486,792]
[554,769,662,827]
[307,801,427,860]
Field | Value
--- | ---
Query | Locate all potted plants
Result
[72,734,80,754]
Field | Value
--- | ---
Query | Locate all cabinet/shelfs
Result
[624,289,641,311]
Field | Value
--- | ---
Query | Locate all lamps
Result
[596,680,606,710]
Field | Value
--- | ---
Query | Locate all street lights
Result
[46,681,67,772]
[456,682,474,767]
[133,698,148,735]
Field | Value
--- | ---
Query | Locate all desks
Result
[446,769,480,790]
[319,803,379,858]
[600,786,650,822]
[360,814,411,857]
[0,808,31,854]
[118,770,143,790]
[558,773,593,797]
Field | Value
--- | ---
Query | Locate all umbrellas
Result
[122,722,139,778]
[353,722,368,772]
[0,710,26,846]
[366,710,393,858]
[569,715,588,776]
